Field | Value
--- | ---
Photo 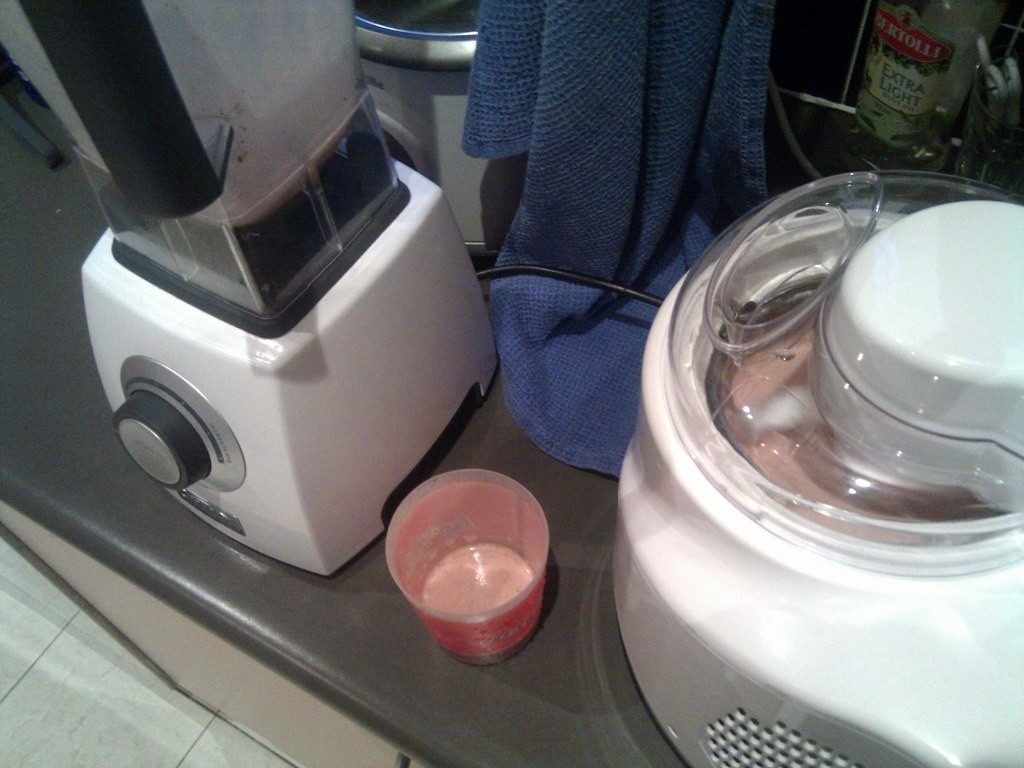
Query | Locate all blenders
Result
[1,1,499,577]
[611,170,1021,768]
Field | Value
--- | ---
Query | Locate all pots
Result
[353,0,530,260]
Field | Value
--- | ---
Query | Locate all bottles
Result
[831,0,1005,185]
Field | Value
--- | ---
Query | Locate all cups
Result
[956,65,1024,196]
[380,465,553,668]
